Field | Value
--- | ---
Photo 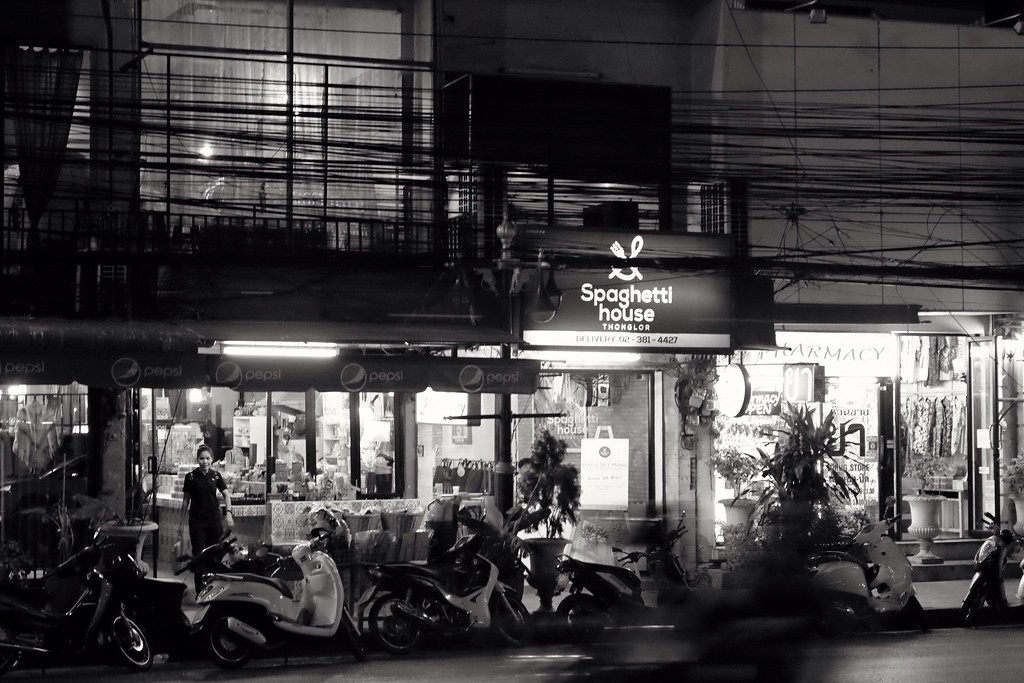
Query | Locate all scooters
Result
[804,496,928,638]
[0,508,367,677]
[353,510,535,655]
[960,511,1024,629]
[554,511,717,643]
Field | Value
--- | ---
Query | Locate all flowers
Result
[901,446,953,496]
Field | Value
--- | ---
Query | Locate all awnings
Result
[0,321,199,389]
[173,319,541,394]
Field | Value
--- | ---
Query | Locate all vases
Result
[902,495,947,565]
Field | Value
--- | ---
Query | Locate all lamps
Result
[222,343,345,361]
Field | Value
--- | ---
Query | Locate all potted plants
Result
[709,401,867,563]
[1000,446,1024,597]
[517,428,582,618]
[75,471,164,558]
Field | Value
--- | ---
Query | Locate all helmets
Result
[308,508,338,538]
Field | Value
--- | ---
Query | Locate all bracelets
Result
[224,509,232,514]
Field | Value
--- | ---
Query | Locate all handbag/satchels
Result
[169,532,191,574]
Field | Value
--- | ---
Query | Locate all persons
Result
[365,440,394,494]
[176,444,235,600]
